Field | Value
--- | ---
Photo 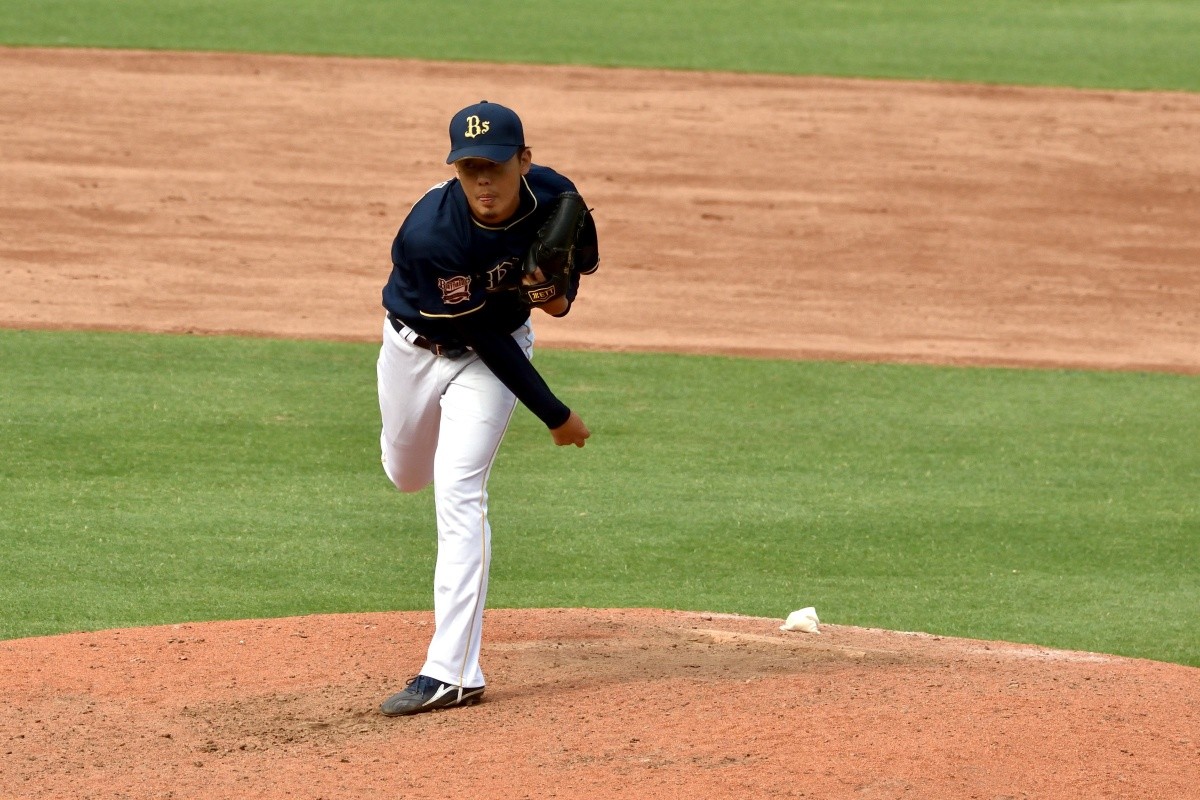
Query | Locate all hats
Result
[446,100,524,165]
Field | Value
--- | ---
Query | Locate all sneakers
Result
[381,675,485,717]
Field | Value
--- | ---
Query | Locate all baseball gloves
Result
[518,192,595,308]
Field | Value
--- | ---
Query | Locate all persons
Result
[375,100,601,719]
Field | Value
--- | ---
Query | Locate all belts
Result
[389,314,470,360]
